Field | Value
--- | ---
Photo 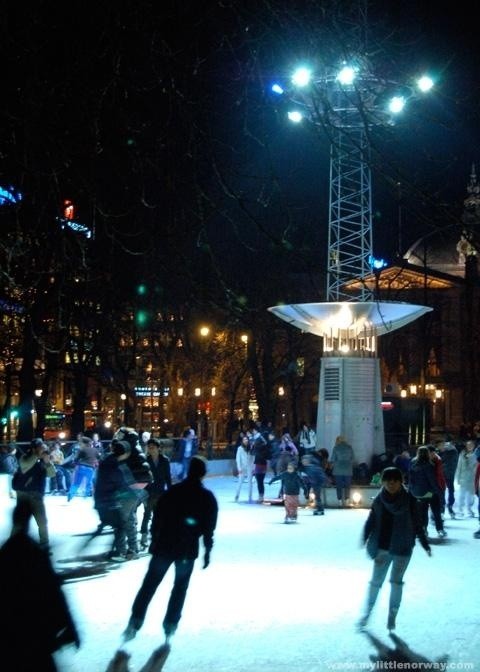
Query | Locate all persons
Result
[0,502,80,672]
[356,466,432,632]
[0,419,473,561]
[122,455,218,637]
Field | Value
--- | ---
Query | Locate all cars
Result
[2,405,258,446]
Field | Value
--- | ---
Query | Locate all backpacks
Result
[351,452,397,487]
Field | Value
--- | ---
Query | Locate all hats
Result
[190,455,207,476]
[382,466,402,480]
[31,437,44,448]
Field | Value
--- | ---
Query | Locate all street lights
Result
[262,6,441,508]
